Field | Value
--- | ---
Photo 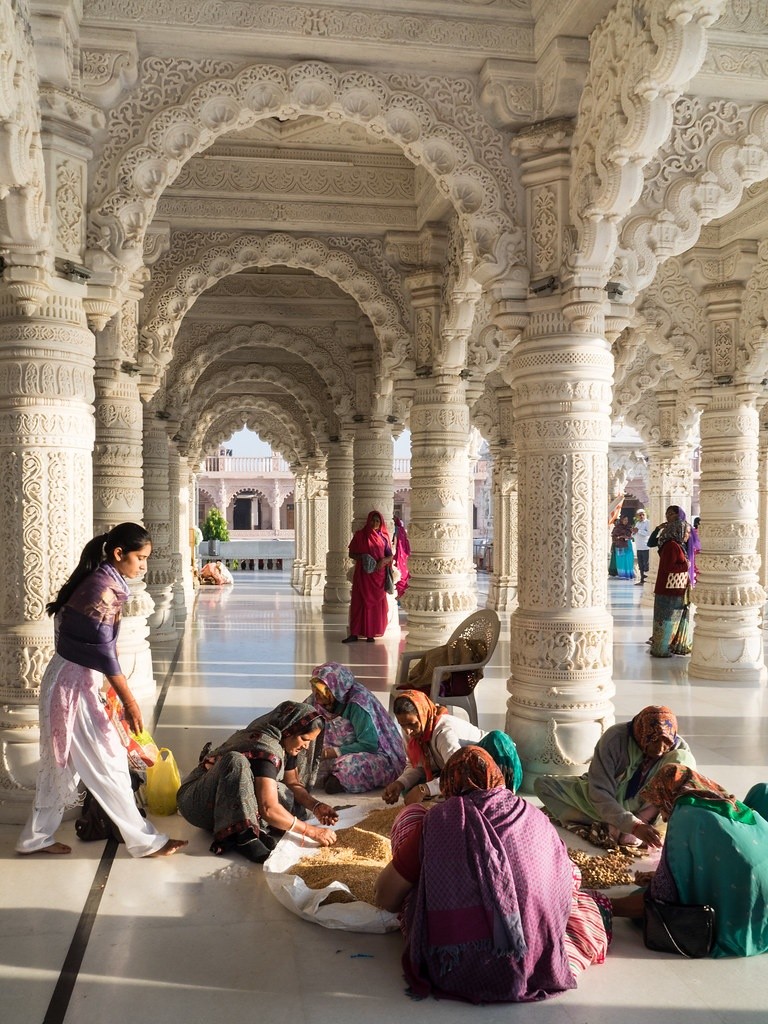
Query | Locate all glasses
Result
[639,514,645,516]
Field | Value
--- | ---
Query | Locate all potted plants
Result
[201,506,229,557]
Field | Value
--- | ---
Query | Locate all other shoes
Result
[342,636,357,644]
[366,637,375,641]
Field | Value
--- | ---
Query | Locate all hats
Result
[636,508,645,514]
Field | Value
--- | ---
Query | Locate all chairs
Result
[388,608,501,728]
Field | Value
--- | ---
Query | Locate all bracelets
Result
[419,784,426,794]
[312,802,321,814]
[287,816,298,833]
[124,699,136,708]
[324,749,329,758]
[301,822,308,847]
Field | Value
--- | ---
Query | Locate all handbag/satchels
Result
[147,748,183,817]
[386,565,394,595]
[642,897,716,958]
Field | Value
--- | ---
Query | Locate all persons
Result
[692,517,702,575]
[341,510,394,643]
[303,663,408,794]
[609,766,768,958]
[608,514,636,580]
[380,690,518,804]
[644,505,702,657]
[534,704,695,848]
[631,509,650,586]
[15,523,189,859]
[392,517,411,600]
[377,747,614,1003]
[176,702,340,862]
